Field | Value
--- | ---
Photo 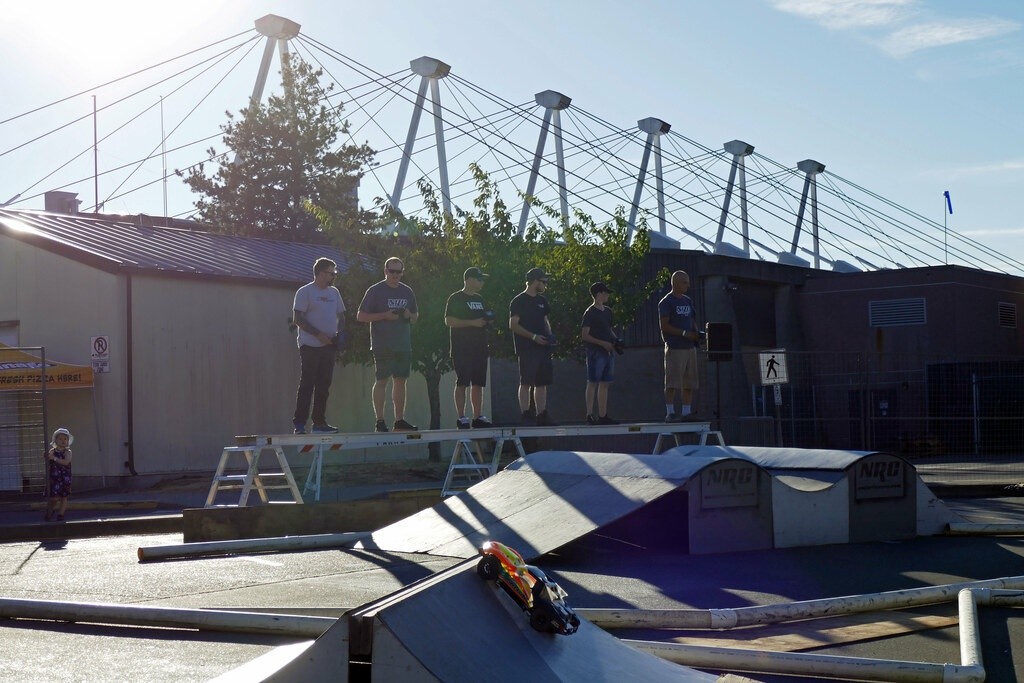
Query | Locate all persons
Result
[44,428,74,522]
[657,271,708,422]
[357,257,419,431]
[580,282,625,425]
[293,258,348,435]
[508,268,560,426]
[444,267,496,429]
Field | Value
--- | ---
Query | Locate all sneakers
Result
[374,420,388,432]
[472,416,493,427]
[392,420,417,431]
[457,418,469,428]
[536,411,560,426]
[521,415,539,425]
[665,413,682,422]
[310,424,338,433]
[294,424,306,433]
[597,413,620,425]
[585,414,609,424]
[680,410,705,421]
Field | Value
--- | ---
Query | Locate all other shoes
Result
[57,514,64,519]
[45,511,55,520]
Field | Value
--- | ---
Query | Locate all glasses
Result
[324,271,337,277]
[387,269,403,274]
[537,279,548,284]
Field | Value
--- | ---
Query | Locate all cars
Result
[476,539,580,636]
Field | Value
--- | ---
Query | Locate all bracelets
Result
[682,330,686,337]
[532,334,536,341]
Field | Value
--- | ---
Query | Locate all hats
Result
[50,428,74,448]
[590,282,613,295]
[464,267,489,278]
[526,268,551,281]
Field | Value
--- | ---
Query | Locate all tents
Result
[0,341,106,490]
